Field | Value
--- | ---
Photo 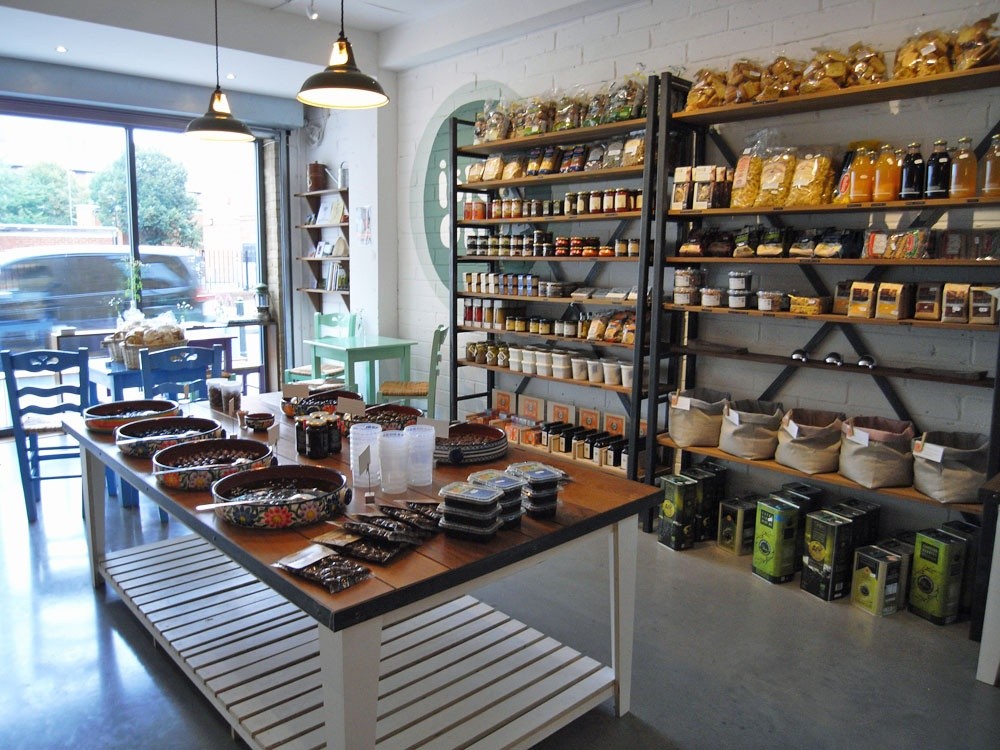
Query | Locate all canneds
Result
[463,273,539,296]
[294,411,341,459]
[537,281,577,298]
[505,315,577,337]
[466,340,517,367]
[463,188,646,221]
[466,231,639,256]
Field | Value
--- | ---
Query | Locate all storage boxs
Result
[653,464,981,627]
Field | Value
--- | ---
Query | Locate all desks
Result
[60,383,670,750]
[302,334,413,406]
[82,351,208,510]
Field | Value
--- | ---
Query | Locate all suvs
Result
[0,250,209,353]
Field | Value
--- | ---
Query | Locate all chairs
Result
[131,341,223,522]
[0,342,119,523]
[283,312,363,383]
[376,323,448,418]
[185,335,238,373]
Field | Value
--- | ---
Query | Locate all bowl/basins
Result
[113,415,227,459]
[82,398,183,436]
[507,343,594,379]
[244,412,275,432]
[280,388,364,418]
[281,397,304,417]
[341,403,424,442]
[436,460,565,544]
[433,422,509,464]
[151,437,278,493]
[209,463,353,531]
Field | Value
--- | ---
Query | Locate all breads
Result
[127,325,184,344]
[684,15,1000,112]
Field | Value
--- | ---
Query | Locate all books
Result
[324,262,351,292]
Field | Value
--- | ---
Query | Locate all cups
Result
[570,356,650,388]
[349,422,436,494]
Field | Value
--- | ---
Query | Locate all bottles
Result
[295,411,342,459]
[848,133,1000,204]
[540,421,647,470]
[466,339,517,368]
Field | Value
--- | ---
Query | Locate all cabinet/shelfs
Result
[642,29,1000,535]
[295,186,350,311]
[445,67,693,479]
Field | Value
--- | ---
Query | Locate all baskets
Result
[103,330,129,360]
[118,334,189,370]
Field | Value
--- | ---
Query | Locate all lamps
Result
[180,0,256,143]
[294,0,391,111]
